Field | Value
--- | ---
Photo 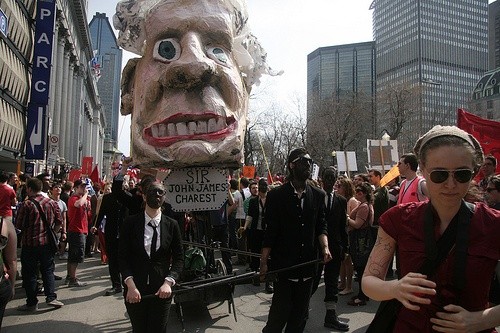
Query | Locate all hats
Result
[413,125,485,165]
[73,179,89,186]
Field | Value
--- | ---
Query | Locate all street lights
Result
[331,150,336,166]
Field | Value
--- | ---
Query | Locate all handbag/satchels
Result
[363,297,404,330]
[46,226,60,254]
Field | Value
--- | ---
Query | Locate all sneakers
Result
[105,286,122,295]
[69,279,87,287]
[348,293,369,305]
[46,299,64,307]
[326,308,350,331]
[17,303,38,312]
[339,288,355,295]
[335,314,350,323]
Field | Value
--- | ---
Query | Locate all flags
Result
[91,49,102,83]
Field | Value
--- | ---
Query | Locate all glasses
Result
[291,156,312,164]
[485,187,498,193]
[421,165,478,183]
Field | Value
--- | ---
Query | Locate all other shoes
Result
[64,276,70,285]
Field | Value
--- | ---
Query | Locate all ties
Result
[262,207,266,233]
[149,223,158,258]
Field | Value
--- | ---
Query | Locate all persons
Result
[85,176,179,297]
[367,168,394,281]
[115,178,185,333]
[336,178,360,296]
[310,165,350,331]
[483,173,500,308]
[397,153,431,205]
[305,169,408,208]
[113,0,284,171]
[15,178,65,312]
[238,176,274,294]
[0,170,50,297]
[64,179,88,287]
[0,216,17,332]
[46,182,67,281]
[361,124,500,333]
[479,154,497,193]
[259,146,333,333]
[181,171,287,271]
[58,180,74,259]
[346,181,374,305]
